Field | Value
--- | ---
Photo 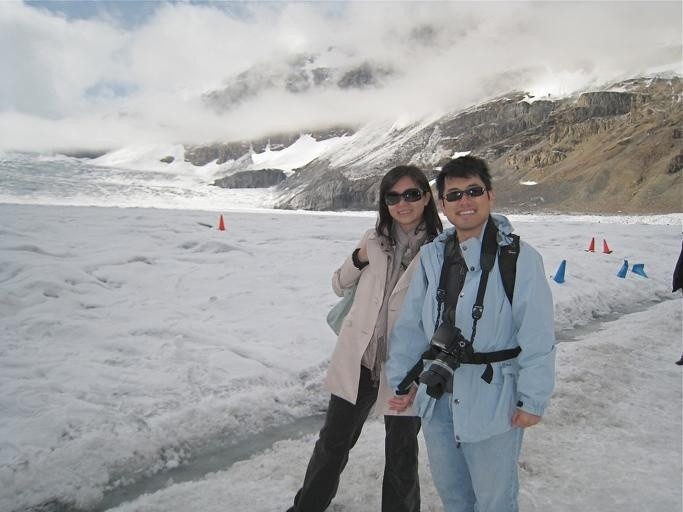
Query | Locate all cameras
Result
[416,323,474,399]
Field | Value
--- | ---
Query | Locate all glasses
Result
[442,186,486,202]
[384,188,426,206]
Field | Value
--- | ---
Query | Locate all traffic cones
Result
[584,237,595,252]
[553,259,568,283]
[217,213,226,231]
[601,239,613,254]
[615,260,629,279]
[631,264,647,278]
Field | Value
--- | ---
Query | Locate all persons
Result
[384,156,557,512]
[272,163,448,512]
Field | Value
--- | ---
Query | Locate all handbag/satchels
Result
[327,284,359,336]
